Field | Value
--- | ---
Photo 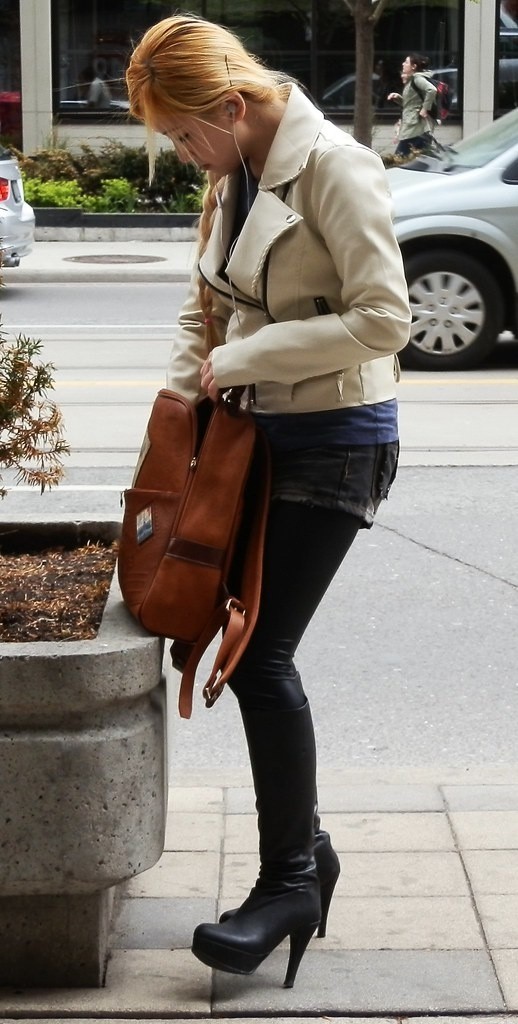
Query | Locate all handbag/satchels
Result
[392,120,402,145]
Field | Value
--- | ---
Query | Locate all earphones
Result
[224,103,236,121]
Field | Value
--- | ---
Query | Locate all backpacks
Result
[118,382,270,720]
[411,72,451,126]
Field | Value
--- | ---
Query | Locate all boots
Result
[217,789,342,939]
[189,694,322,988]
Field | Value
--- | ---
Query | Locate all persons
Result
[386,52,439,156]
[88,57,112,108]
[121,15,417,993]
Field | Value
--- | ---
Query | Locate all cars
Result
[319,27,518,122]
[0,142,37,268]
[383,105,517,371]
